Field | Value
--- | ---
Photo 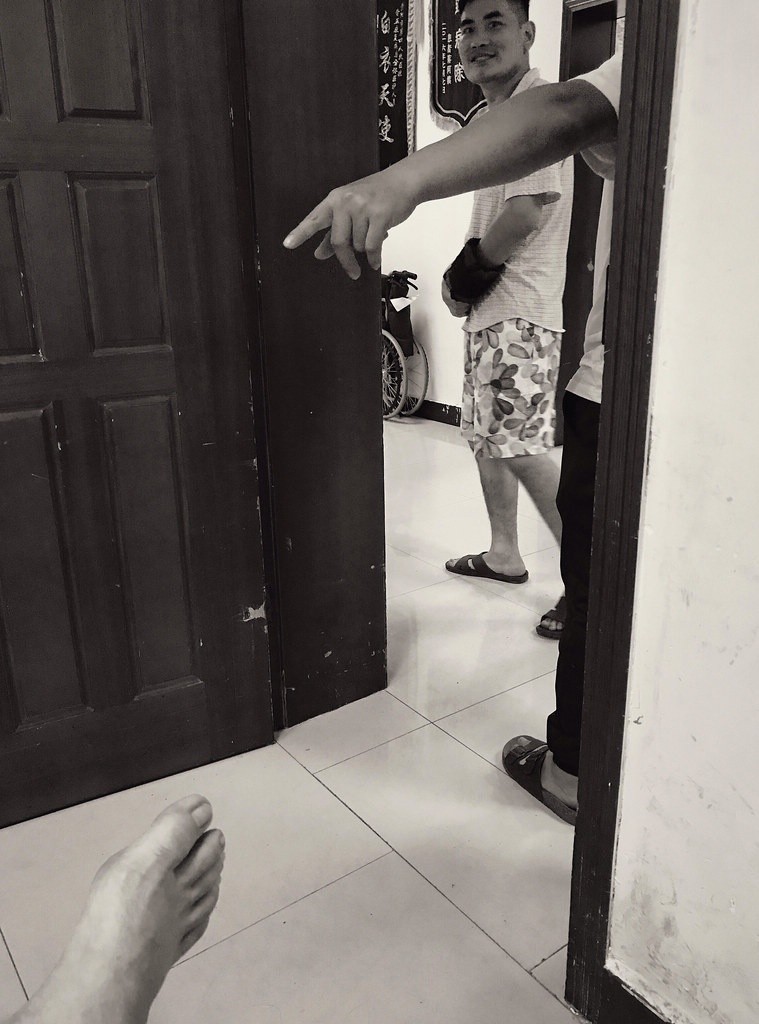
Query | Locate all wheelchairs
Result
[379,270,430,420]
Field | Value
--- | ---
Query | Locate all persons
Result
[0,794,224,1024]
[283,48,624,826]
[442,1,573,638]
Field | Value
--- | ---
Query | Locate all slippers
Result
[537,596,568,640]
[445,551,528,584]
[502,734,577,826]
